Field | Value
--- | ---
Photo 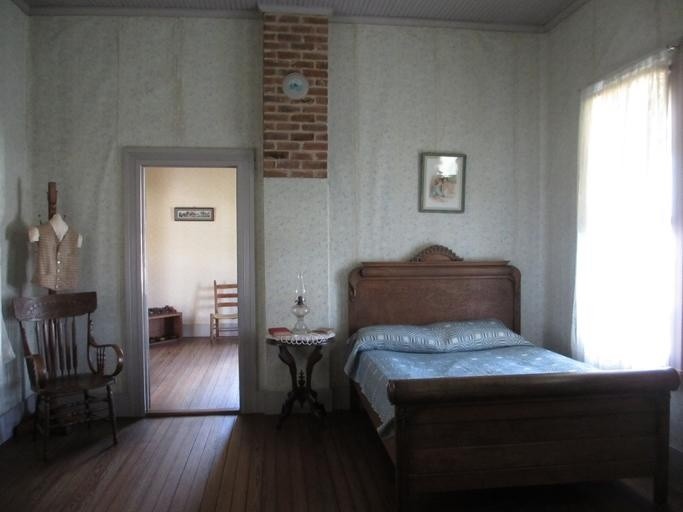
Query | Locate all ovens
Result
[348,245,681,512]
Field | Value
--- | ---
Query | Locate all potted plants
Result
[174,207,214,222]
[418,152,466,214]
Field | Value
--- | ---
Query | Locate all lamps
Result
[209,281,238,344]
[12,291,125,460]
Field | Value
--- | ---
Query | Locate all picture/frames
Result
[266,332,335,436]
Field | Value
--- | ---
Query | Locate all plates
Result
[149,312,184,347]
